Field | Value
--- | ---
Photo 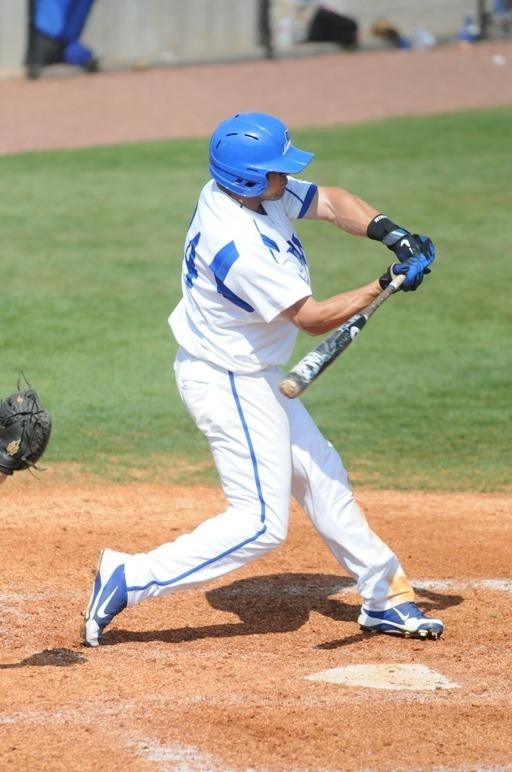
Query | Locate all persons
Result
[0,390,51,484]
[81,110,444,649]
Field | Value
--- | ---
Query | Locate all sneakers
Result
[356,601,445,641]
[82,546,127,649]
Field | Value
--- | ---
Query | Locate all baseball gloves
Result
[0,370,52,478]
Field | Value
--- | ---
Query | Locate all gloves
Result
[366,211,436,276]
[378,255,426,293]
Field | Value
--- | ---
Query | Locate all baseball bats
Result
[280,274,404,399]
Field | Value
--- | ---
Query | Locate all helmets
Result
[208,110,316,199]
